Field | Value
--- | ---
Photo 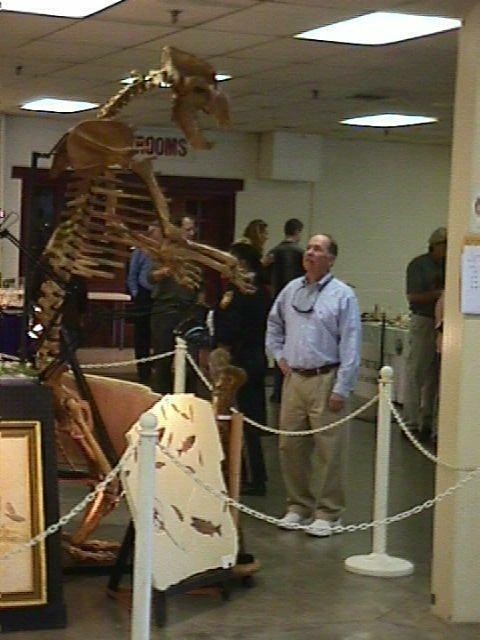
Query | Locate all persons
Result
[232,219,275,270]
[215,242,272,497]
[265,232,363,538]
[400,227,447,443]
[429,290,445,444]
[127,226,163,384]
[147,216,207,395]
[266,219,306,403]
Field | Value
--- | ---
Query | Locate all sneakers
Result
[421,430,436,445]
[276,510,311,529]
[238,479,268,498]
[400,427,423,443]
[302,517,346,537]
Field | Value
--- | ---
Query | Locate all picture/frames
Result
[0,420,48,607]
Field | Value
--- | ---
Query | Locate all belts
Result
[287,360,342,378]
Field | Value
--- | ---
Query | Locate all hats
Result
[427,223,447,245]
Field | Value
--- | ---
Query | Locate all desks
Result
[83,290,132,351]
[350,320,414,424]
